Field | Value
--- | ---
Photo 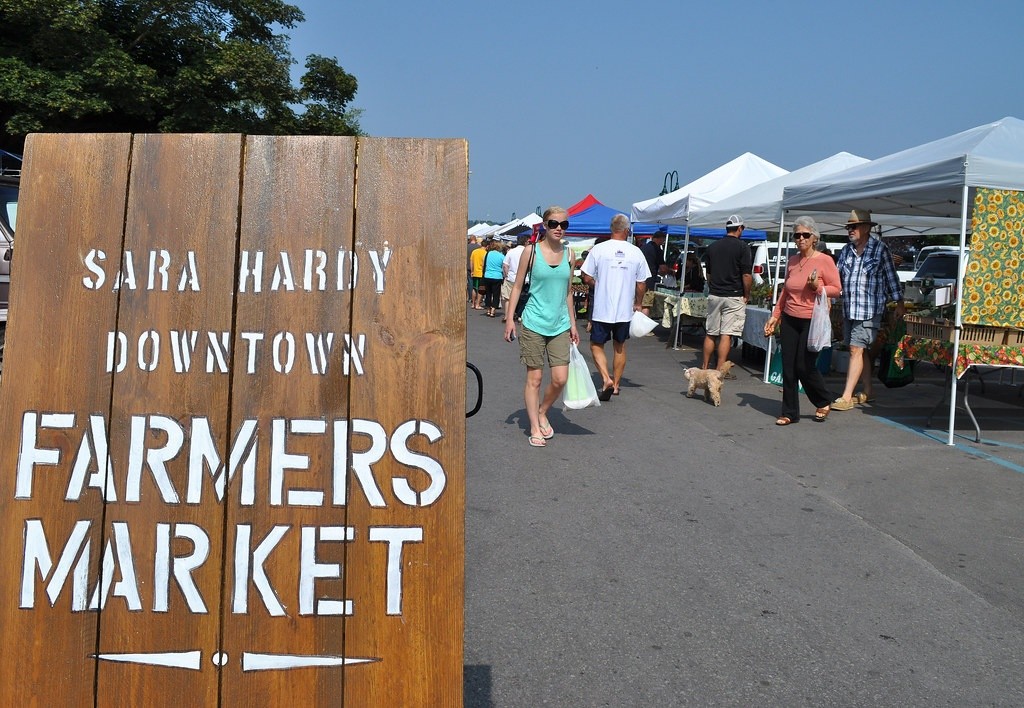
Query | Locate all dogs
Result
[684,361,735,407]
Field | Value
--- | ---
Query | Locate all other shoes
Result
[851,393,875,403]
[831,397,853,410]
[723,372,737,379]
[469,301,507,321]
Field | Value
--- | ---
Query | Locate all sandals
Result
[815,405,830,419]
[776,417,798,425]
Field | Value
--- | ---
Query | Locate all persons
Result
[701,215,752,380]
[763,208,906,426]
[581,214,652,395]
[505,207,579,446]
[467,230,699,337]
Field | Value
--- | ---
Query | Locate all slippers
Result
[529,436,546,447]
[612,388,619,395]
[599,386,614,402]
[539,415,554,439]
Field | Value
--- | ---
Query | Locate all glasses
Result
[844,225,861,230]
[741,226,745,230]
[793,232,813,239]
[545,220,570,230]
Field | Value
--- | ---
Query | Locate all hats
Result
[725,215,745,228]
[844,208,878,227]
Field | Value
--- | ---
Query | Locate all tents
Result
[467,194,631,248]
[632,115,1024,222]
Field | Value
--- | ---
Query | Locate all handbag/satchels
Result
[515,244,535,317]
[806,287,832,352]
[563,342,601,411]
[877,317,915,388]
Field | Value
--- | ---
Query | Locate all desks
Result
[895,335,1024,444]
[741,305,781,384]
[647,290,706,348]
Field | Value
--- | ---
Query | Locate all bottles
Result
[703,281,709,297]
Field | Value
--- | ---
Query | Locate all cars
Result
[660,239,707,291]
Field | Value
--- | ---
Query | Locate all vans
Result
[749,241,969,302]
